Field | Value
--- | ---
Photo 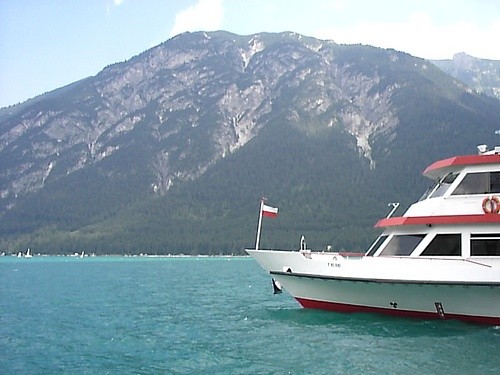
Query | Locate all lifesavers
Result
[481,195,500,214]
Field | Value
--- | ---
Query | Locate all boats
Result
[245,129,499,327]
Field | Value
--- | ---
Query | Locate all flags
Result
[262,203,278,218]
